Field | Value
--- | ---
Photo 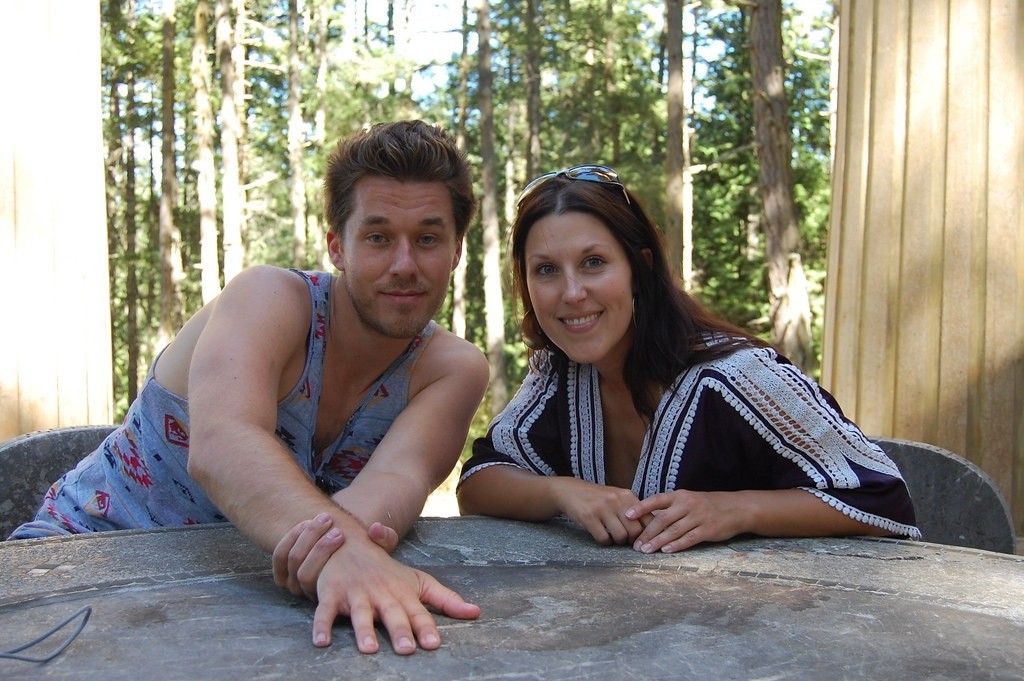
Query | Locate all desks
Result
[0,516,1024,681]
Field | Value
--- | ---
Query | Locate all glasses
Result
[517,166,634,212]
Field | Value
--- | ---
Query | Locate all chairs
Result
[0,424,122,541]
[867,435,1016,554]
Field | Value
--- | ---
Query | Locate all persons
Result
[455,165,924,555]
[7,122,492,656]
